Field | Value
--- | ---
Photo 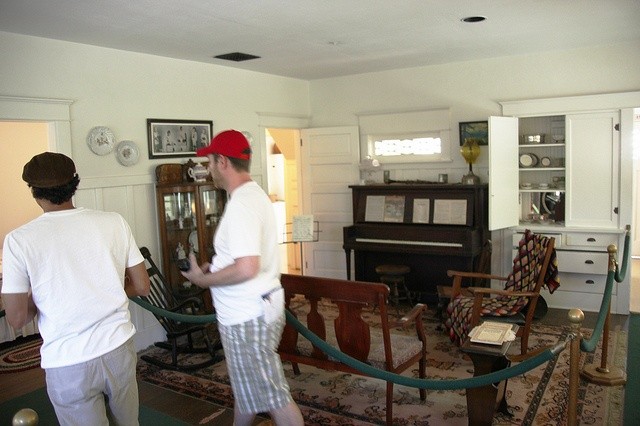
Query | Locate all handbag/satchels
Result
[261,288,285,324]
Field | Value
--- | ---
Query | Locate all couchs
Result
[278,273,428,424]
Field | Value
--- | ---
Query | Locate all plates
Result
[539,187,549,190]
[519,152,553,167]
[188,231,198,254]
[87,126,115,156]
[116,141,142,166]
[553,135,565,140]
[521,187,532,190]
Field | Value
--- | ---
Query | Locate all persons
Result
[179,130,306,426]
[166,126,208,152]
[1,152,149,426]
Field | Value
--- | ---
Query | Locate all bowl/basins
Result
[522,183,531,186]
[522,133,545,144]
[539,184,547,187]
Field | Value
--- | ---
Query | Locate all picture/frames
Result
[458,121,488,146]
[147,118,213,158]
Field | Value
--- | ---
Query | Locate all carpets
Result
[137,294,627,426]
[1,333,43,374]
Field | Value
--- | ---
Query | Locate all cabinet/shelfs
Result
[501,228,619,315]
[160,180,228,338]
[487,110,622,233]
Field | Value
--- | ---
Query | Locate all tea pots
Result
[188,161,209,183]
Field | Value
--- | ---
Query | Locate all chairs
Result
[435,239,492,331]
[445,228,561,363]
[125,246,226,372]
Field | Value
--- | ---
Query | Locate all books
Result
[434,198,468,225]
[365,194,405,223]
[469,321,514,346]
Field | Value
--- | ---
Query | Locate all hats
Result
[22,152,76,187]
[196,130,250,160]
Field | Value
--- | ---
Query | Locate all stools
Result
[371,264,413,316]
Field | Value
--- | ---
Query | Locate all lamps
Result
[460,140,481,186]
